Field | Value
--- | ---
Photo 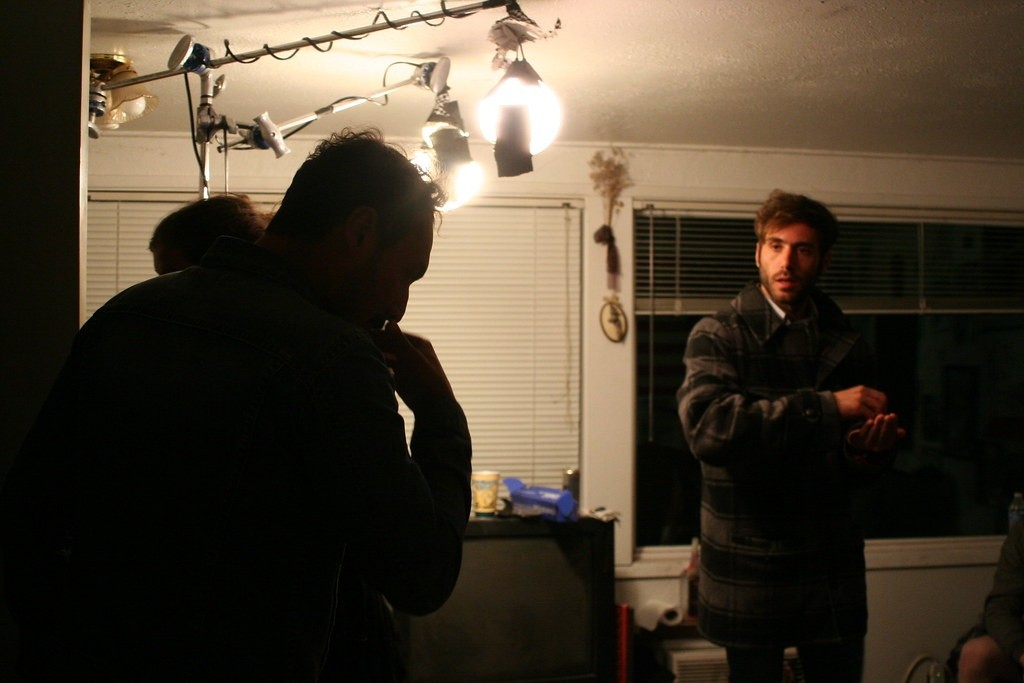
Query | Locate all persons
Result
[0,137,472,683]
[676,192,905,683]
[957,515,1024,683]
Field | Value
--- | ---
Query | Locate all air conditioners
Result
[667,648,800,683]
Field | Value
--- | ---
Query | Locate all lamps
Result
[167,34,487,212]
[89,0,565,177]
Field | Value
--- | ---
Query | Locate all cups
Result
[471,470,500,513]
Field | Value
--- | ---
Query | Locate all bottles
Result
[1008,493,1024,534]
[679,538,701,620]
